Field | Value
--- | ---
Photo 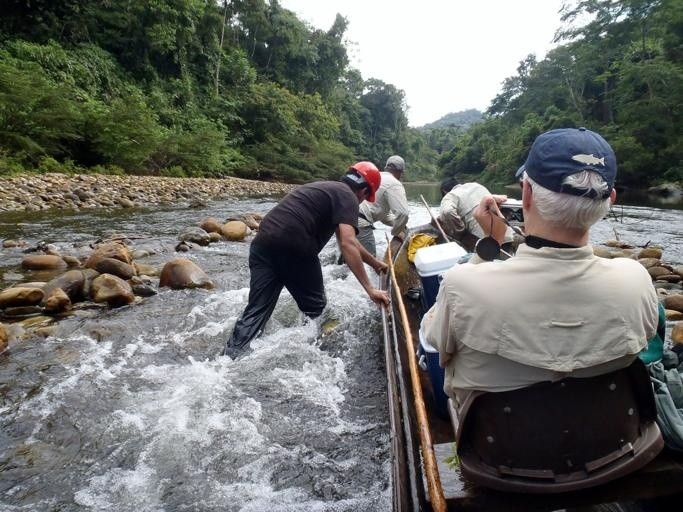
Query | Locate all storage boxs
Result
[414,240,469,423]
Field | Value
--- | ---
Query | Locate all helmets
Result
[345,161,382,205]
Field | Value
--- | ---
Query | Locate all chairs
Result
[444,353,666,493]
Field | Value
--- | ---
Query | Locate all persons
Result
[218,160,391,369]
[435,178,514,255]
[415,126,665,428]
[335,155,409,267]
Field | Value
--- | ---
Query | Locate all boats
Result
[378,223,683,511]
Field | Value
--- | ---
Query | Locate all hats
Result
[439,176,460,199]
[512,126,615,200]
[383,155,407,172]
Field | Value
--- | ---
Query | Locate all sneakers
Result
[319,316,345,338]
